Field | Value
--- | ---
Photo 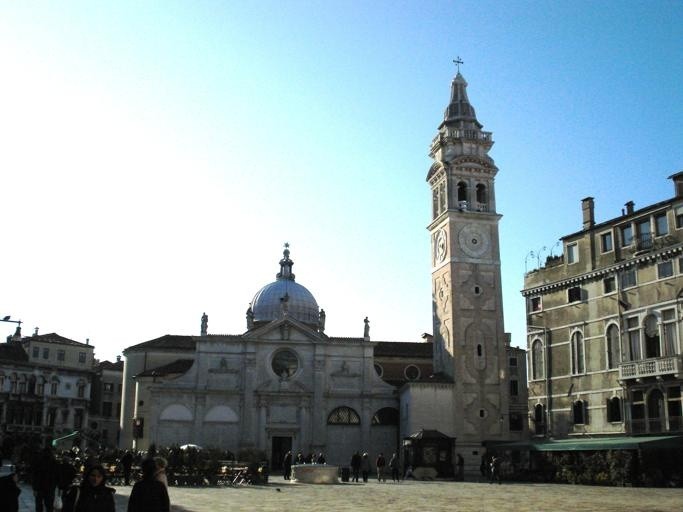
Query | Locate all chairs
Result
[68,462,257,485]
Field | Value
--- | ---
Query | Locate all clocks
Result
[458,223,489,258]
[433,229,448,263]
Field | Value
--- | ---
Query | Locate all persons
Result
[310,454,317,466]
[295,453,305,465]
[350,451,361,482]
[0,443,208,511]
[376,453,385,481]
[362,316,368,336]
[318,309,324,328]
[282,448,292,482]
[489,456,500,485]
[316,452,325,464]
[360,453,368,483]
[454,453,463,481]
[200,313,207,332]
[387,452,398,482]
